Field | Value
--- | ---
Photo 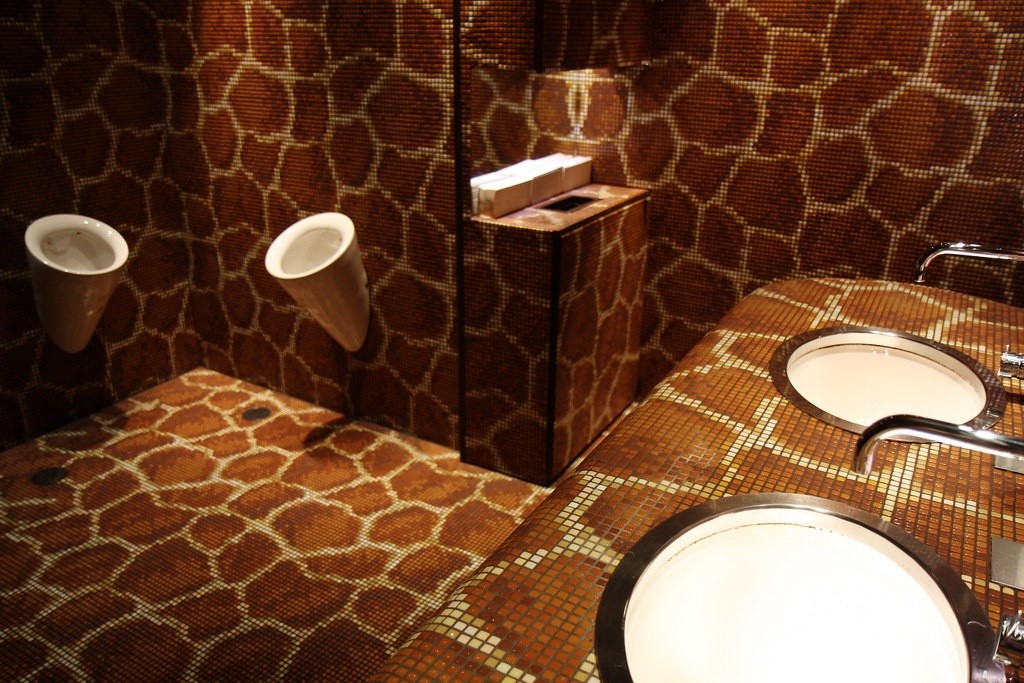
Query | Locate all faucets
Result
[913,242,1024,283]
[850,413,1024,475]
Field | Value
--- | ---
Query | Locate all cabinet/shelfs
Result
[463,181,652,484]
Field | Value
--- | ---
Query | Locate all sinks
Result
[769,324,1009,444]
[593,490,1009,682]
